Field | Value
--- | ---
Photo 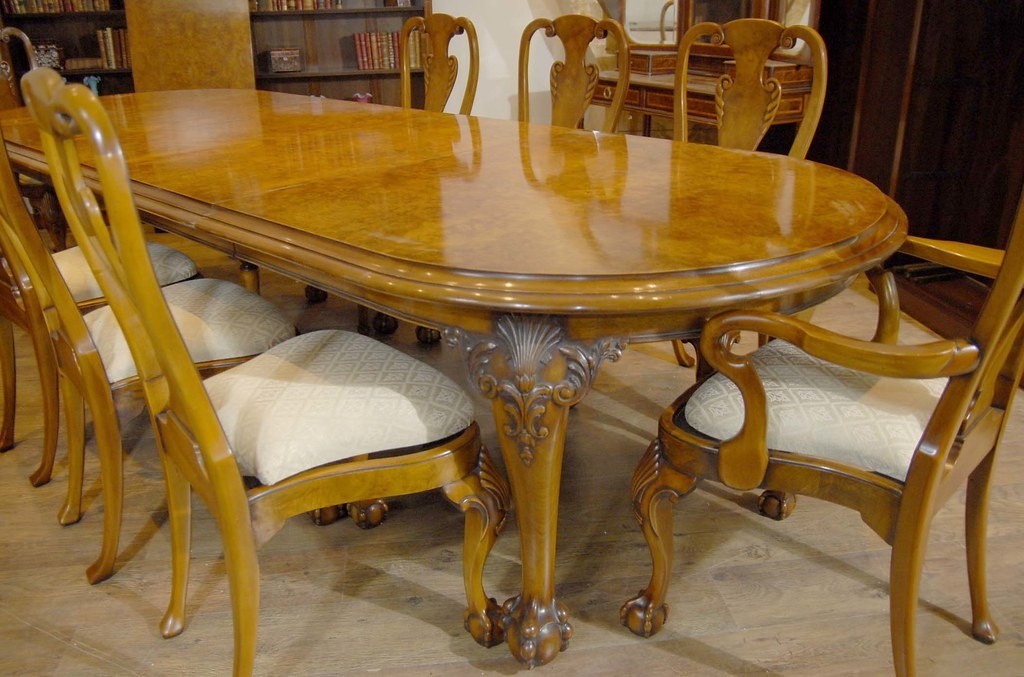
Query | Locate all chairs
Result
[299,15,823,354]
[618,182,1024,676]
[0,66,509,677]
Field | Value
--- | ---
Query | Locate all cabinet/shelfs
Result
[0,0,434,118]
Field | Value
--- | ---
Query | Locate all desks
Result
[0,84,912,667]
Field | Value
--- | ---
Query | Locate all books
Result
[353,30,420,69]
[0,0,110,13]
[65,27,131,70]
[383,0,411,8]
[249,0,343,12]
